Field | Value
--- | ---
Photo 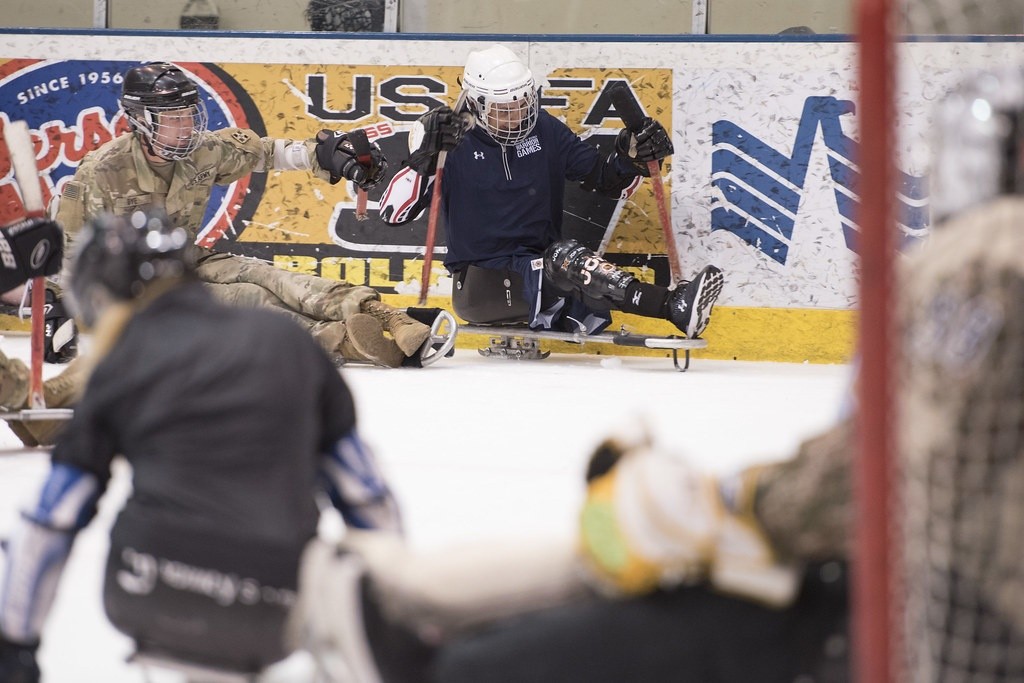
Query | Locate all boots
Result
[339,313,405,368]
[360,300,432,357]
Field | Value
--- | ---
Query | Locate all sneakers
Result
[9,355,96,447]
[669,265,724,339]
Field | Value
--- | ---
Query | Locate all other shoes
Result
[298,535,457,683]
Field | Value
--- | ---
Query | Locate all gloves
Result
[0,217,64,296]
[316,129,389,192]
[0,631,40,683]
[614,120,674,177]
[44,288,79,364]
[408,105,463,176]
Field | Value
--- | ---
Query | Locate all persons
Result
[294,74,1024,683]
[404,44,725,340]
[53,60,432,369]
[0,202,410,683]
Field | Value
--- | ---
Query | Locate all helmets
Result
[462,44,535,130]
[121,61,199,146]
[62,207,190,330]
